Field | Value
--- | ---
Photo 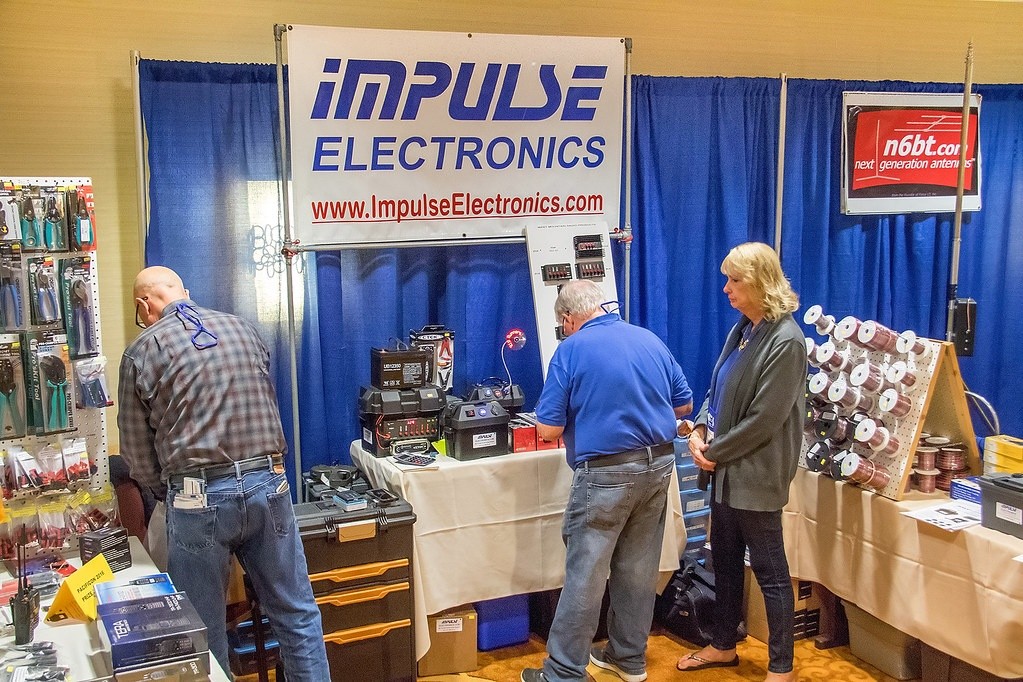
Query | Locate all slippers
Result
[677,650,739,671]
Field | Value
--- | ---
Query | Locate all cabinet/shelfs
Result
[292,488,417,682]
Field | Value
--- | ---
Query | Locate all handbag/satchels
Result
[661,558,747,646]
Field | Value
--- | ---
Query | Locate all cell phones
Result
[366,488,399,502]
[336,490,363,502]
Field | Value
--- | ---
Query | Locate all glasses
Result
[136,297,148,329]
[562,310,571,337]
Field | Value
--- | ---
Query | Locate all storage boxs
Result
[950,477,982,505]
[982,435,1023,480]
[473,594,530,651]
[507,411,565,453]
[417,604,478,677]
[840,600,1010,682]
[978,472,1023,541]
[80,526,211,682]
[741,565,827,645]
[671,438,712,565]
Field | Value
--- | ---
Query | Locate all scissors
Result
[47,198,66,248]
[22,197,40,247]
[75,197,94,248]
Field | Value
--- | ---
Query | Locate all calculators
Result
[393,452,436,466]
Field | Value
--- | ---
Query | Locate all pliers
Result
[40,356,67,429]
[34,270,58,321]
[1,262,22,326]
[0,360,23,439]
[72,279,96,352]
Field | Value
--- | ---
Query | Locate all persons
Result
[676,242,807,682]
[521,280,693,682]
[117,266,332,682]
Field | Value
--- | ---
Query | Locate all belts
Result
[589,442,674,467]
[171,455,285,482]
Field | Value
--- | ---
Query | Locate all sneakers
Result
[521,667,549,682]
[590,646,647,682]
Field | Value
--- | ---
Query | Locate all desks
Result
[350,437,688,664]
[0,535,233,682]
[781,465,1023,680]
